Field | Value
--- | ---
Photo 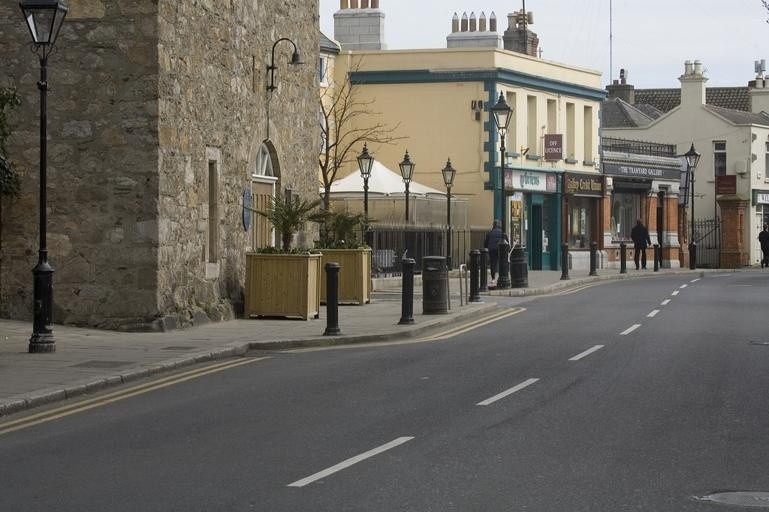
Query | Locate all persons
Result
[483,219,509,284]
[631,219,651,269]
[758,223,769,269]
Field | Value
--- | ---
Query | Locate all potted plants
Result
[236,185,374,323]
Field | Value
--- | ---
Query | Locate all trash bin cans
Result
[422,256,447,315]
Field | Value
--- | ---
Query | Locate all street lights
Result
[356,141,374,246]
[490,89,514,286]
[19,1,69,350]
[685,142,701,269]
[398,148,415,224]
[442,157,457,269]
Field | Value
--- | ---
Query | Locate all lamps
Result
[266,37,306,93]
